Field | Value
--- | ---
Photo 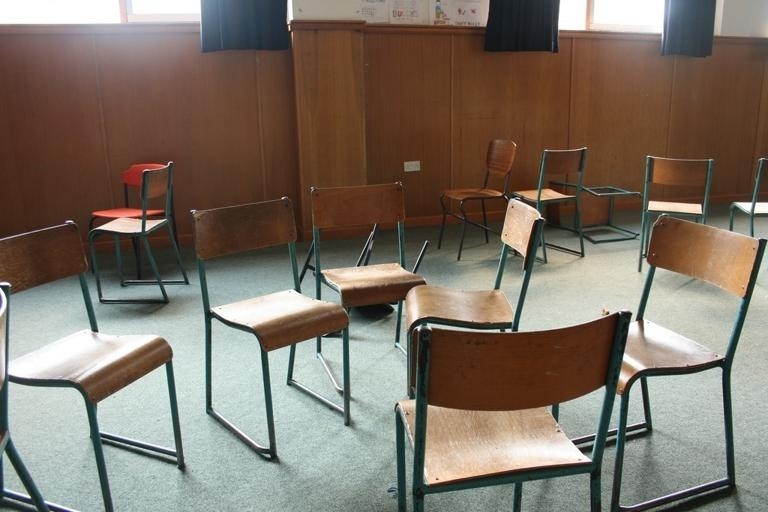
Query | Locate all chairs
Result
[550,212,767,512]
[190,197,354,464]
[87,160,190,303]
[405,199,543,400]
[640,155,713,273]
[730,156,767,243]
[550,180,639,244]
[436,138,519,262]
[311,181,426,397]
[395,310,630,511]
[511,147,586,263]
